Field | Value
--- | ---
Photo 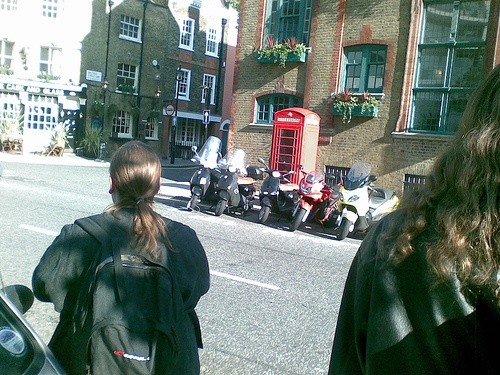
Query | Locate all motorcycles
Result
[288,164,341,232]
[257,157,300,225]
[208,146,264,217]
[187,135,228,213]
[335,162,402,241]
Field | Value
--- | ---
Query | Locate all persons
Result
[32,140,210,375]
[329,63,500,375]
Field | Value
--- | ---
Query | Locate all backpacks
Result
[61,213,201,375]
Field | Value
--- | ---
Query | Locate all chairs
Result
[42,142,66,157]
[2,136,24,154]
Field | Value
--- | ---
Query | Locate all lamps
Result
[102,78,109,90]
[154,88,163,97]
